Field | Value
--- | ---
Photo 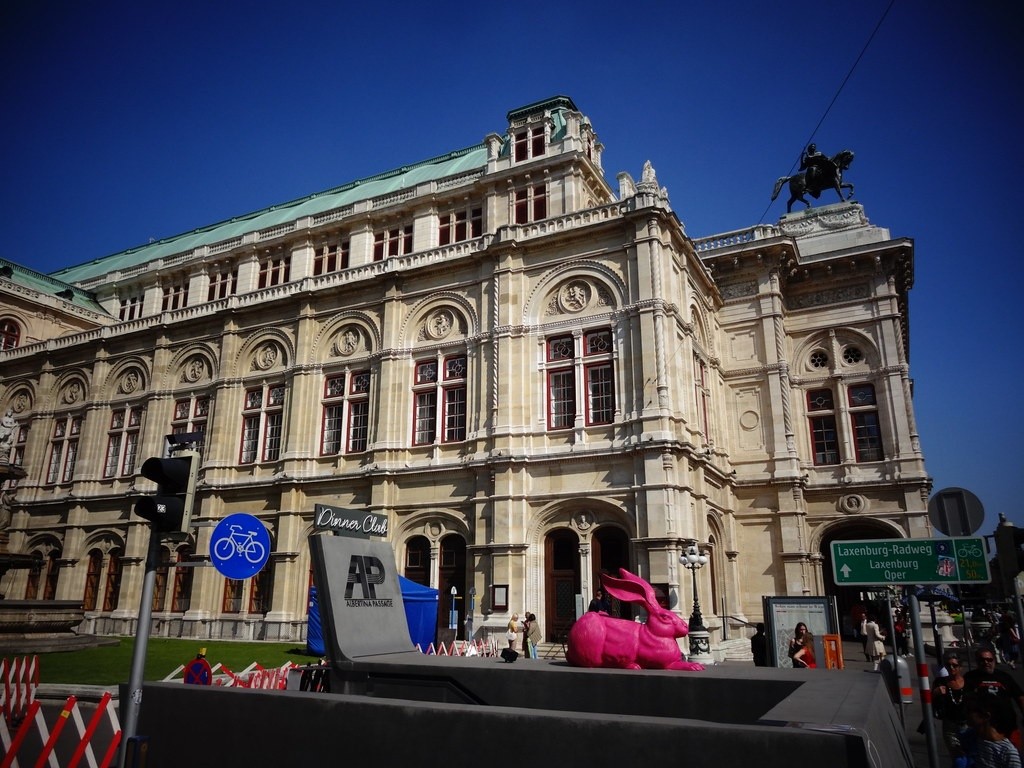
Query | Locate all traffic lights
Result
[129,447,202,535]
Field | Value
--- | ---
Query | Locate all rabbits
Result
[565,567,707,673]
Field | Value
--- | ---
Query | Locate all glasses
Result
[980,657,994,662]
[945,664,959,670]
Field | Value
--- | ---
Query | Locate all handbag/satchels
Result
[895,625,902,633]
[505,628,517,641]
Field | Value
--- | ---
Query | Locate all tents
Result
[306,573,440,655]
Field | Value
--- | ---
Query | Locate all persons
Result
[798,143,828,184]
[587,590,607,613]
[860,614,886,671]
[750,623,768,667]
[931,612,1024,768]
[507,612,542,659]
[789,622,815,669]
[895,608,915,659]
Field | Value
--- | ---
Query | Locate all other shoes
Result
[907,653,914,658]
[902,654,907,658]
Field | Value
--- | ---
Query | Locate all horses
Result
[771,148,856,215]
